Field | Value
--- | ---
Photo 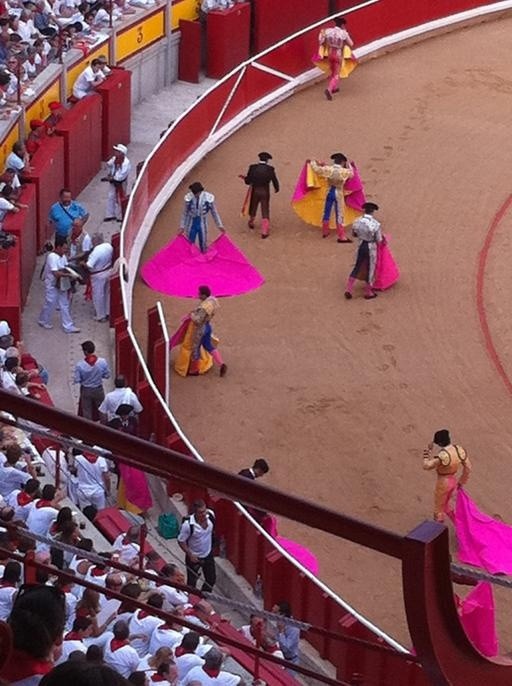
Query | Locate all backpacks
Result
[181,511,216,546]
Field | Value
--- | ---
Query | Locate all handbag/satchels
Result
[55,275,71,292]
[157,512,180,540]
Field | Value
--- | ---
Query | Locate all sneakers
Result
[38,321,53,329]
[64,327,80,333]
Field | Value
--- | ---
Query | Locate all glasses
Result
[6,583,66,624]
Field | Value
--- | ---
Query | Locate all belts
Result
[90,264,111,274]
[284,654,297,661]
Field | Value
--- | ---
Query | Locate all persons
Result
[344,203,386,299]
[311,153,353,242]
[1,1,306,686]
[423,428,472,526]
[318,15,353,99]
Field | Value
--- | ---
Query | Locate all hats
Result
[48,101,61,111]
[30,119,42,129]
[434,429,449,443]
[98,55,107,62]
[330,153,346,160]
[334,18,346,24]
[113,144,128,155]
[258,152,272,159]
[23,1,36,5]
[362,202,379,210]
[115,404,134,416]
[189,181,204,191]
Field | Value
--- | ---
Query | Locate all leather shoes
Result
[220,363,228,377]
[332,88,340,93]
[365,292,377,300]
[322,232,331,238]
[104,216,116,221]
[188,371,199,376]
[324,89,331,100]
[248,221,254,229]
[337,238,354,243]
[344,291,353,299]
[261,233,270,239]
